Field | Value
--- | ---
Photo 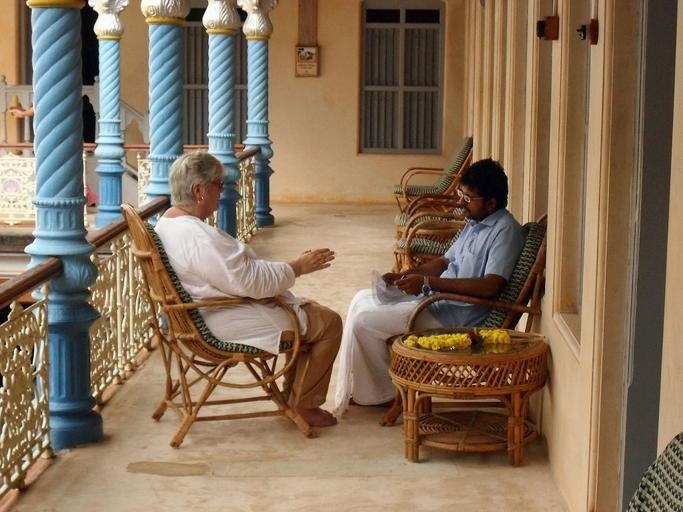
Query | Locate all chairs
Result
[120,204,314,448]
[382,134,550,428]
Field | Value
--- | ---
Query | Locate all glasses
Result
[219,180,224,187]
[457,189,483,203]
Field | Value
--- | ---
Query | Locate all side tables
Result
[388,327,550,466]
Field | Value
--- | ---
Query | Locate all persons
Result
[328,157,524,418]
[153,152,343,427]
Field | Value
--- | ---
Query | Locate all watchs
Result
[422,276,430,296]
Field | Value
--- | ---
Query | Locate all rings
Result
[320,259,324,264]
